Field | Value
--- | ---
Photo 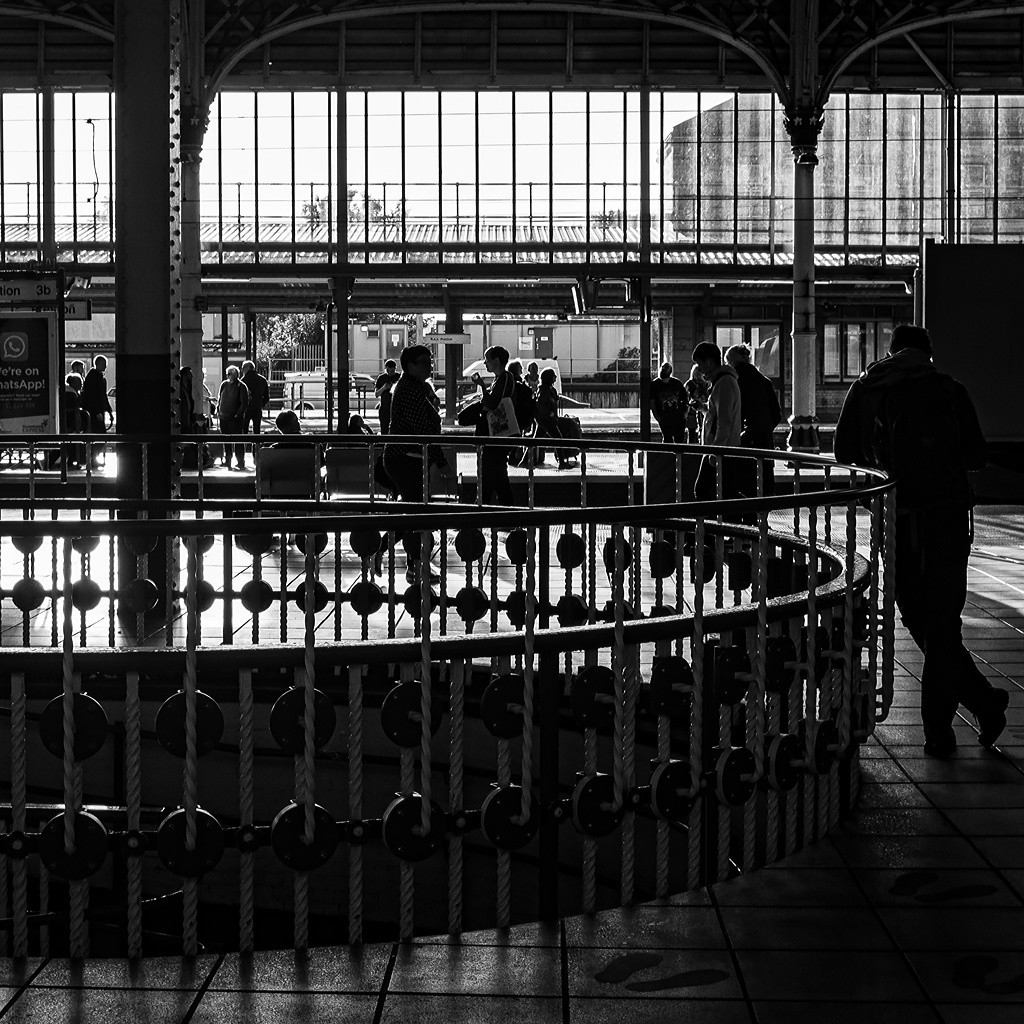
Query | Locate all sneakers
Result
[923,727,957,754]
[972,687,1010,746]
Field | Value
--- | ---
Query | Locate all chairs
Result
[253,440,464,504]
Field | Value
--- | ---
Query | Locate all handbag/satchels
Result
[487,371,522,437]
[456,400,482,426]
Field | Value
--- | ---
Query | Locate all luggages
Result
[556,414,582,465]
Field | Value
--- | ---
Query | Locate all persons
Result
[504,358,576,469]
[267,410,325,469]
[220,365,248,471]
[375,360,402,435]
[39,356,85,471]
[657,341,784,550]
[830,323,1013,762]
[470,345,519,533]
[179,367,204,441]
[81,356,114,469]
[239,360,271,455]
[366,344,457,583]
[347,415,373,442]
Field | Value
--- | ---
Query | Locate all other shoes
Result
[406,560,442,586]
[369,551,383,577]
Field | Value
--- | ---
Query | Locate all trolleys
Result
[527,397,583,464]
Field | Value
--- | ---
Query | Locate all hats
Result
[888,324,934,357]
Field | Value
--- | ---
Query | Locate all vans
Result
[280,369,382,411]
[435,356,562,419]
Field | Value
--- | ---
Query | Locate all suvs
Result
[106,387,116,412]
